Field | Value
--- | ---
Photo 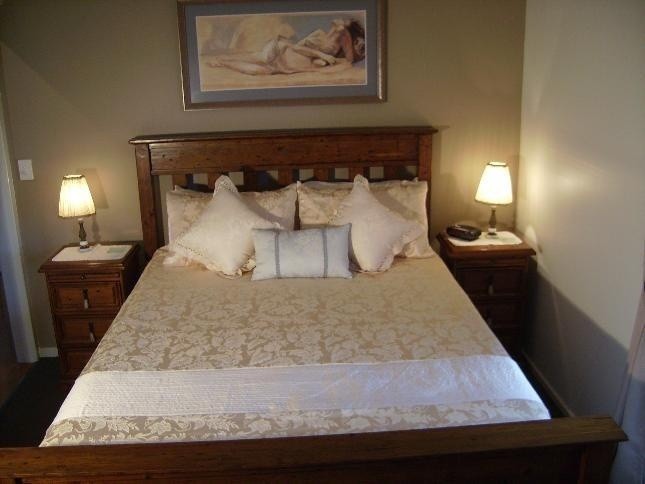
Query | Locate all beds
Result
[1,123,631,483]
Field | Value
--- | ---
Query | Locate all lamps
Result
[475,160,513,239]
[56,174,97,250]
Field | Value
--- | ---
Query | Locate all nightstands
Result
[435,229,537,361]
[38,240,139,384]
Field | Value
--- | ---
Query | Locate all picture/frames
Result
[176,0,389,112]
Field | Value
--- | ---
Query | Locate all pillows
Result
[163,172,436,283]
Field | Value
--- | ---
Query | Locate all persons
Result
[203,18,365,75]
